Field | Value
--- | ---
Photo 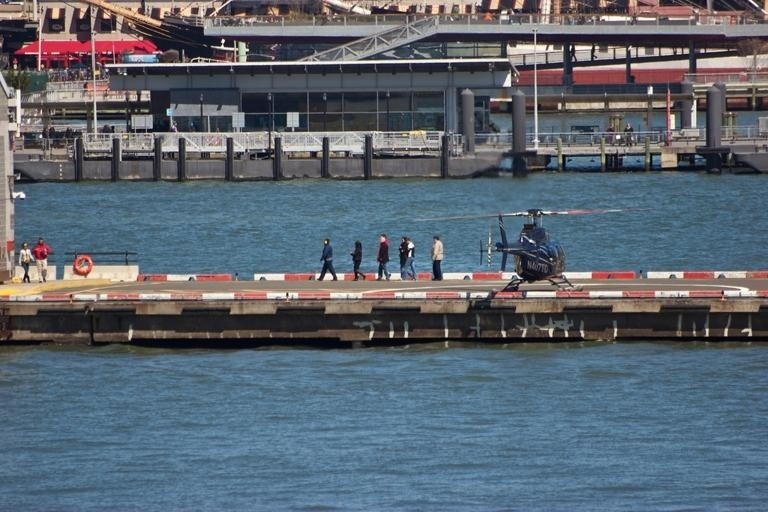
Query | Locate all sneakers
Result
[375,273,391,281]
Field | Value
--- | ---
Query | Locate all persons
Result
[317,238,337,281]
[350,240,366,280]
[606,124,615,144]
[431,236,443,281]
[376,234,391,280]
[399,237,417,280]
[19,242,35,283]
[170,121,177,132]
[624,123,633,146]
[32,237,53,283]
[41,127,72,150]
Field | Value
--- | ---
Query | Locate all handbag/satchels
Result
[353,256,360,261]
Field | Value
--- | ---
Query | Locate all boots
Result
[317,276,323,281]
[23,276,30,283]
[330,277,338,281]
[352,272,366,281]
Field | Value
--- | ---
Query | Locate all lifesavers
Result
[74,254,92,274]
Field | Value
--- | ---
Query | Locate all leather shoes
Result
[432,276,442,281]
[39,279,46,283]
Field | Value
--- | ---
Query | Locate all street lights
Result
[531,28,541,150]
[90,30,97,139]
[36,4,44,72]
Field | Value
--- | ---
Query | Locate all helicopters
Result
[413,208,653,292]
